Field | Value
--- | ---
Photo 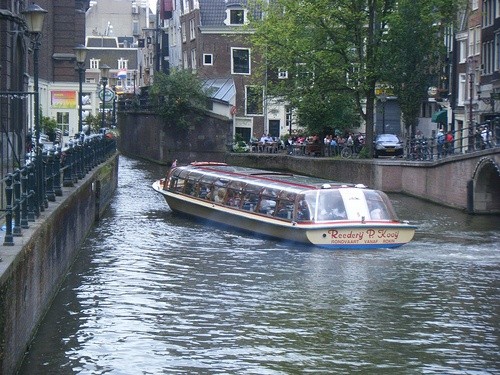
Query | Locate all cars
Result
[372,133,404,159]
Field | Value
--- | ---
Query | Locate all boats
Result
[152,159,419,250]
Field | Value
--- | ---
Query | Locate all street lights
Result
[71,43,89,141]
[380,95,388,134]
[465,55,477,152]
[20,4,47,157]
[108,74,119,128]
[288,99,294,136]
[99,63,111,134]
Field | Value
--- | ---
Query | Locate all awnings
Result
[432,111,446,127]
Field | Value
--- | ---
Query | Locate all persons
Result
[184,181,372,221]
[286,133,319,156]
[251,133,282,153]
[479,126,495,150]
[324,132,366,156]
[415,129,424,161]
[437,130,452,159]
